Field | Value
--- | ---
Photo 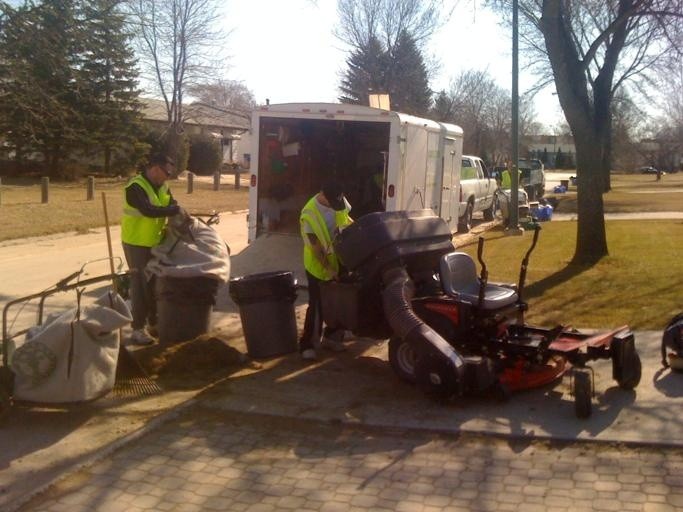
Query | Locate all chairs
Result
[439,252,519,310]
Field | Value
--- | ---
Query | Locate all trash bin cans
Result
[228,270,299,357]
[560,180,569,191]
[524,184,535,202]
[154,272,217,344]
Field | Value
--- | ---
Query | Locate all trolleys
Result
[0,255,127,420]
[495,185,531,229]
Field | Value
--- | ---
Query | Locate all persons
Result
[296,179,356,360]
[120,153,190,344]
[498,161,524,190]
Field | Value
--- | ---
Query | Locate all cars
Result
[637,167,666,176]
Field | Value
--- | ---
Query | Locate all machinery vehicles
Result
[337,209,644,417]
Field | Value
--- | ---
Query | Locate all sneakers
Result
[130,325,156,346]
[301,348,318,362]
[320,336,348,352]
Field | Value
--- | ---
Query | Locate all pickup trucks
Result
[457,155,500,232]
[493,158,545,203]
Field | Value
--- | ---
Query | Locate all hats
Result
[322,180,347,212]
[149,153,175,177]
